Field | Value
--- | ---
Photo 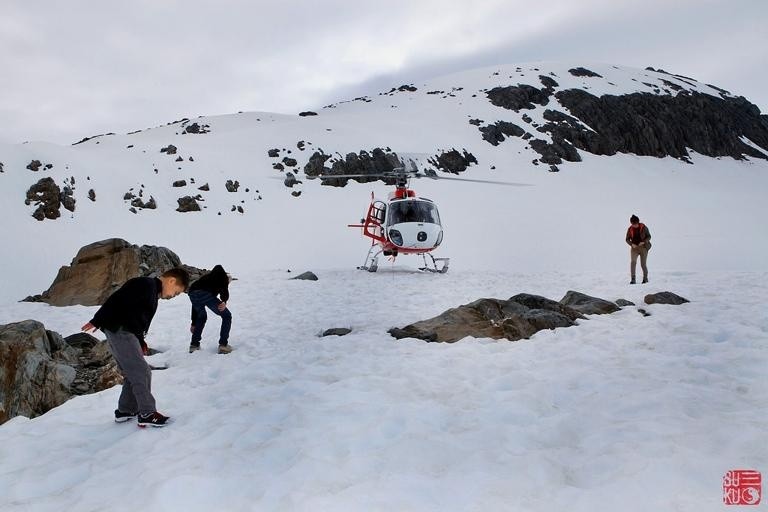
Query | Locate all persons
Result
[189,264,236,354]
[624,214,652,285]
[81,266,189,428]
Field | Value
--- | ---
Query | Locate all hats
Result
[630,215,639,222]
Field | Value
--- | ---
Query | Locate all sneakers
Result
[189,344,201,353]
[114,409,139,423]
[218,344,232,353]
[138,411,171,427]
[630,279,648,284]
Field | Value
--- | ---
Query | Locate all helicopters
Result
[262,166,538,275]
